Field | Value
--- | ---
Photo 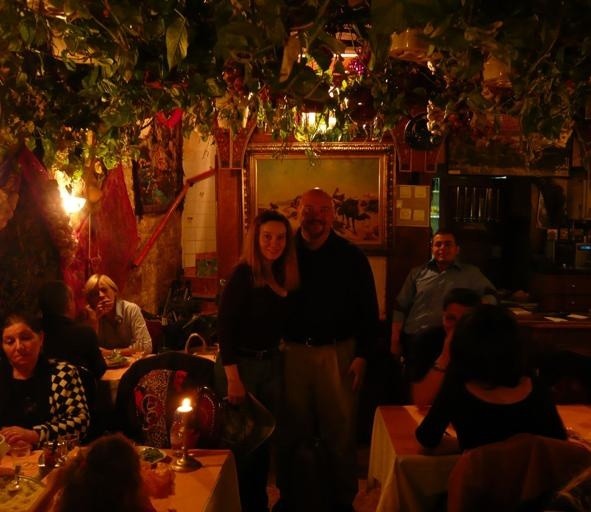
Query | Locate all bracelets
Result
[432,362,448,373]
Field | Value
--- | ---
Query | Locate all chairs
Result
[0,306,591,511]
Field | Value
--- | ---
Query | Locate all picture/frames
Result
[241,142,396,257]
[444,128,576,180]
[132,112,186,217]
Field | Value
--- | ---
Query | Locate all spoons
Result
[6,464,22,491]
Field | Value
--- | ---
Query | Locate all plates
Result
[137,445,168,468]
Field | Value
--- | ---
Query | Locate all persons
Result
[415,305,567,457]
[403,289,484,404]
[210,210,300,511]
[86,273,153,357]
[35,282,105,399]
[390,229,495,364]
[283,188,378,512]
[0,309,90,450]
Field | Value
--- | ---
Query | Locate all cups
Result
[0,436,81,480]
